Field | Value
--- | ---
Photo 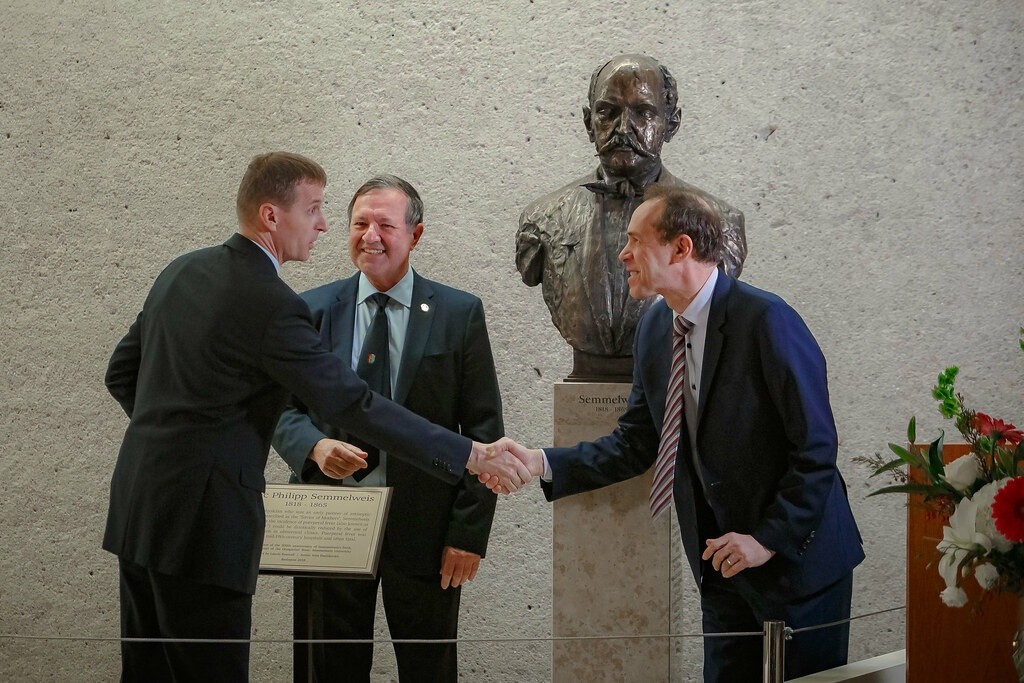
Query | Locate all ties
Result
[348,293,390,482]
[650,315,694,520]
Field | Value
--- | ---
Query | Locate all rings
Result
[726,559,733,565]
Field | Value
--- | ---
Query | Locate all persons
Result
[271,176,505,683]
[469,184,866,683]
[515,54,748,357]
[101,150,532,683]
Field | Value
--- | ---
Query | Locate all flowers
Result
[850,367,1023,667]
